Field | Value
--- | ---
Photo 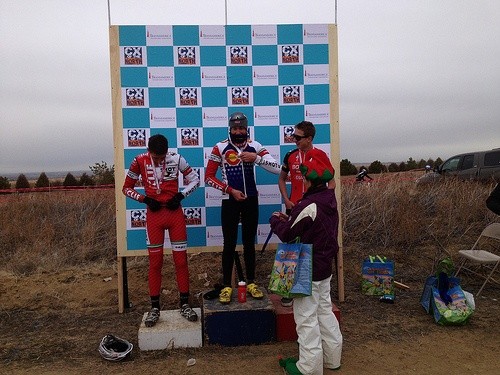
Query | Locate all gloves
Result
[166,192,184,211]
[143,196,162,212]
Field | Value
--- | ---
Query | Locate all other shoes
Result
[281,297,294,306]
[286,362,302,375]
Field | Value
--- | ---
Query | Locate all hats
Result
[300,149,335,185]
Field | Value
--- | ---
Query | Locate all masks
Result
[230,132,248,144]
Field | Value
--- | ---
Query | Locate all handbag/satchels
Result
[430,277,474,327]
[420,258,454,314]
[361,255,395,296]
[268,237,313,299]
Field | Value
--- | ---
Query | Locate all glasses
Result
[292,134,308,141]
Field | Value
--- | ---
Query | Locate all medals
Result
[156,189,161,194]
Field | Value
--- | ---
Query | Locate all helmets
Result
[228,112,248,131]
[98,334,133,362]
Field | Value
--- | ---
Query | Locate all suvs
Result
[415,148,500,186]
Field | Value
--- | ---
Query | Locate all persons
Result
[204,112,282,303]
[356,169,374,181]
[279,120,336,215]
[121,134,200,328]
[269,149,342,375]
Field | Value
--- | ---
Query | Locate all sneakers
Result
[218,287,232,305]
[180,304,198,321]
[145,308,160,327]
[246,283,263,298]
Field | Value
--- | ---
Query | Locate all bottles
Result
[237,281,247,303]
